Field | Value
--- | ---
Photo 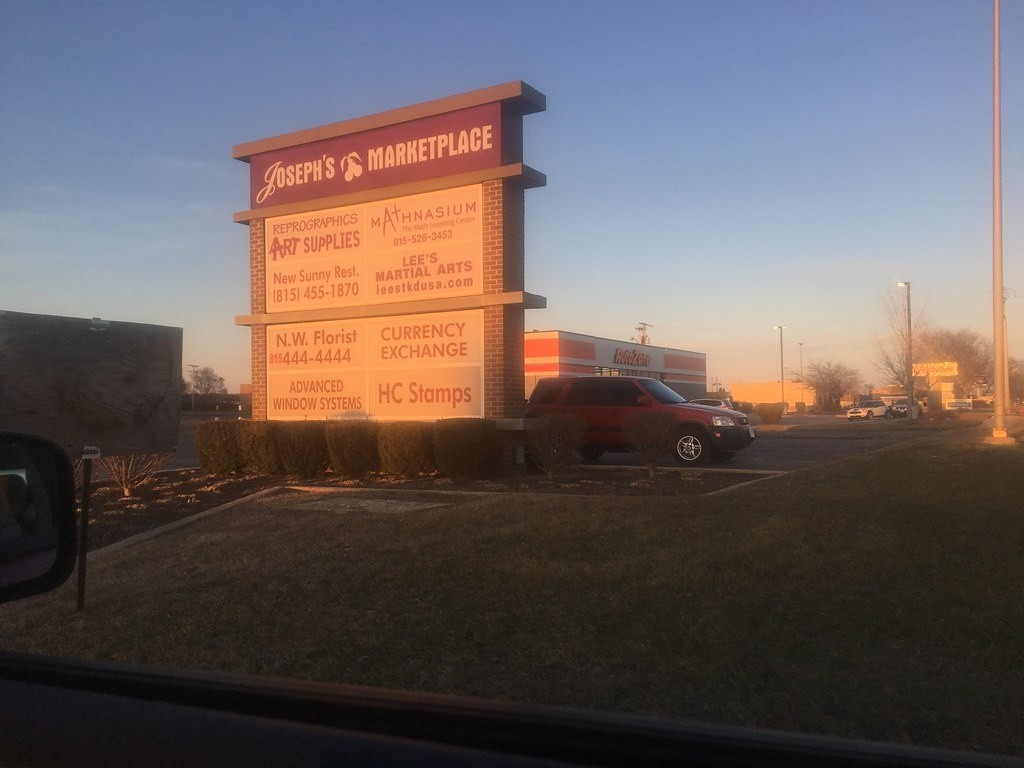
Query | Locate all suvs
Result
[522,374,758,466]
[715,447,717,450]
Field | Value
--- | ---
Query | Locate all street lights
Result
[897,282,914,405]
[774,325,788,402]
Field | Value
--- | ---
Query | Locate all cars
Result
[846,400,891,420]
[688,399,734,410]
[891,398,923,417]
